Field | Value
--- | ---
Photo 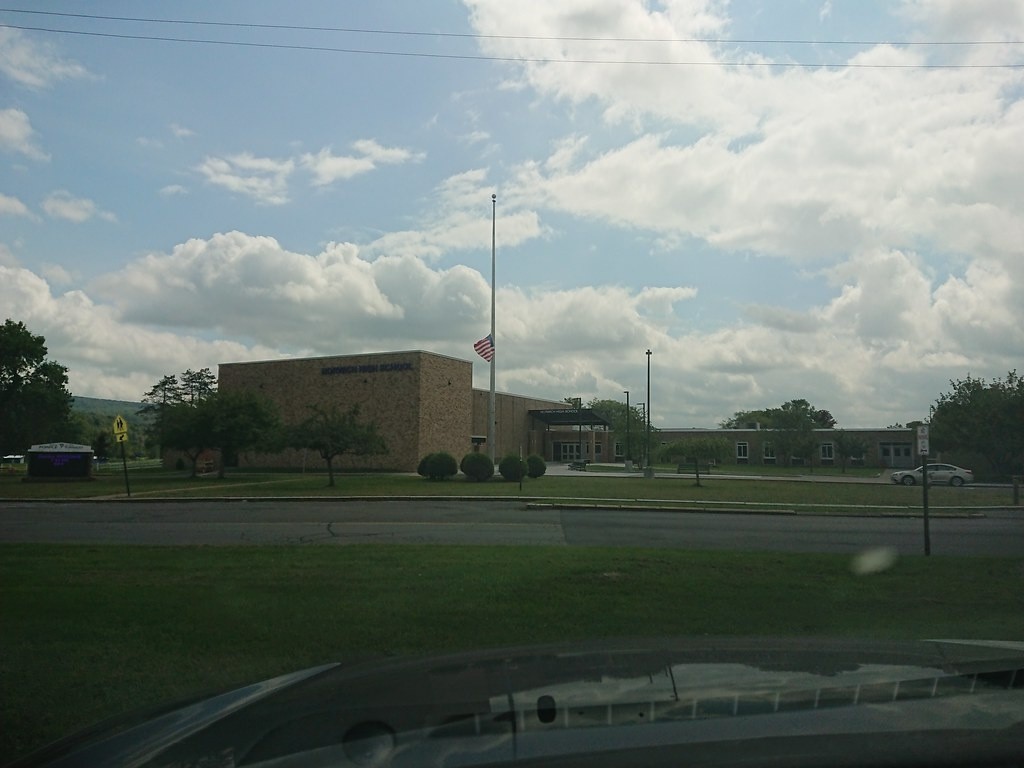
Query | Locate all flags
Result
[475,333,496,363]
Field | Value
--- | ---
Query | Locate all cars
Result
[891,462,974,487]
[201,461,215,473]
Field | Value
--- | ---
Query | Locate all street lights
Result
[637,402,646,464]
[572,397,582,459]
[623,391,630,460]
[645,350,652,466]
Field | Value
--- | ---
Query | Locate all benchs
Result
[568,459,587,472]
[677,464,711,474]
